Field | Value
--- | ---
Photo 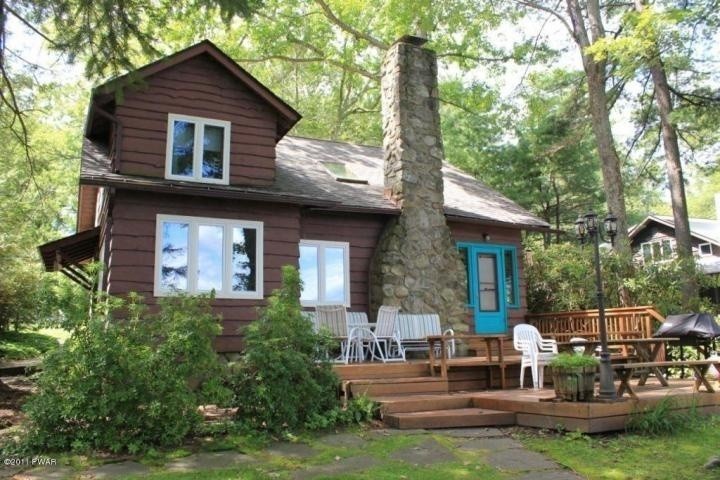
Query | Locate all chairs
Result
[513,324,558,389]
[312,302,406,364]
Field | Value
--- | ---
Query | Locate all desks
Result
[426,332,507,363]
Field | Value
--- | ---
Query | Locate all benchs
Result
[610,358,720,397]
[557,336,681,387]
[302,311,456,359]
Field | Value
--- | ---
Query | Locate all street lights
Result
[576,207,617,399]
